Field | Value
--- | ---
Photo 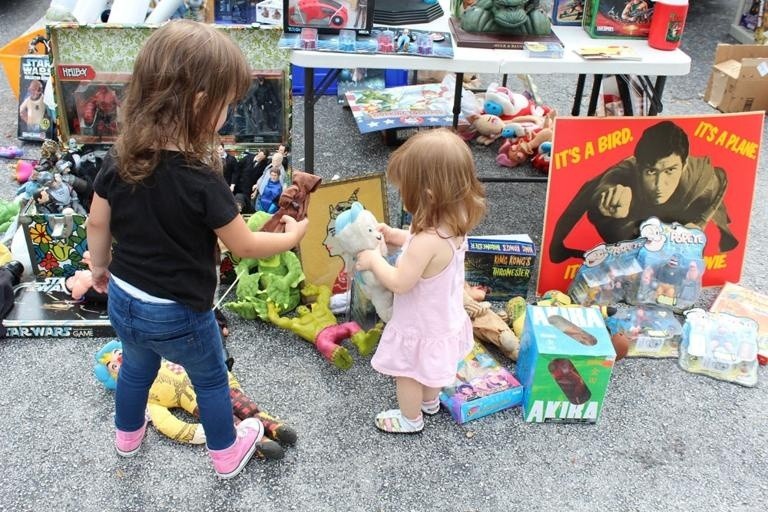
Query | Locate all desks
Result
[285,0,692,197]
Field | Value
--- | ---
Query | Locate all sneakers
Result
[111,408,152,459]
[204,415,266,482]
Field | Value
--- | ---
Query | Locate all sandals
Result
[372,408,429,436]
[420,400,443,417]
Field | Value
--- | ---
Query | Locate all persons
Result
[459,358,510,401]
[67,26,308,479]
[0,79,400,461]
[357,127,475,435]
[549,121,739,264]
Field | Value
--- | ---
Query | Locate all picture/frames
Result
[292,170,388,307]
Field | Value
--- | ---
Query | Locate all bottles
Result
[647,1,691,52]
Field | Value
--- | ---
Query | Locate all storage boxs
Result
[464,232,533,298]
[704,36,767,117]
[0,27,47,101]
[519,303,618,425]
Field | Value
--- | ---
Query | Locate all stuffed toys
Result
[467,280,616,359]
[457,79,556,175]
